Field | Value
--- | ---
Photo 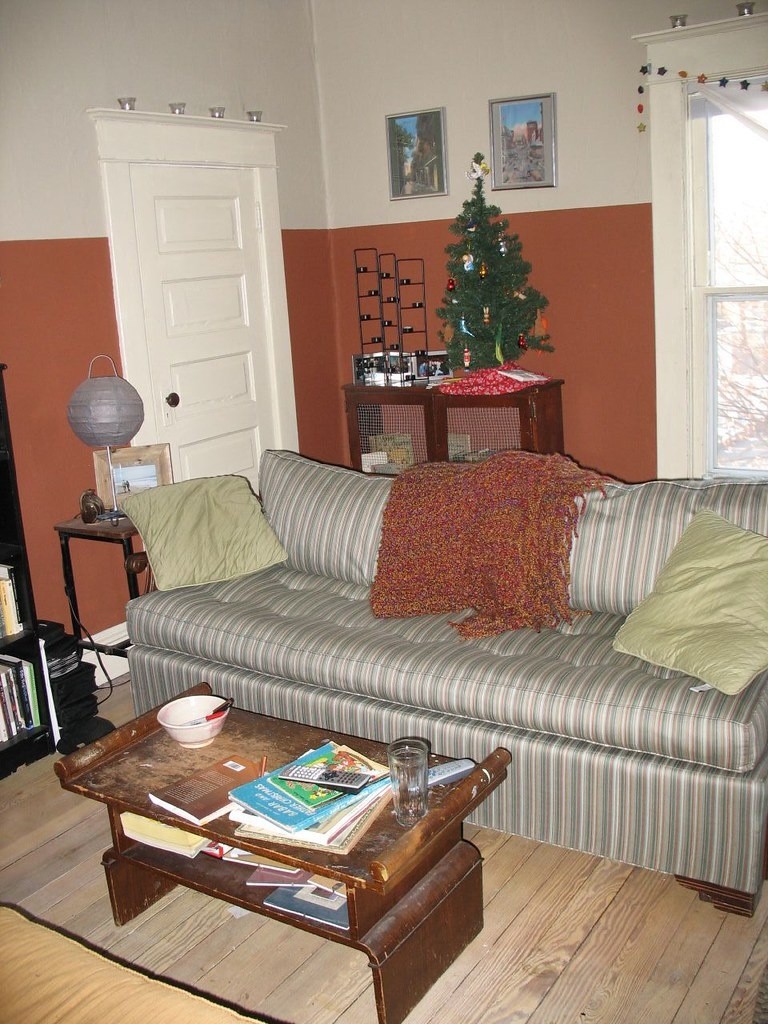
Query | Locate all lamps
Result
[65,353,144,521]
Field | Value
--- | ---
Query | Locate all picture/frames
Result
[384,105,450,201]
[487,91,559,191]
[92,442,173,508]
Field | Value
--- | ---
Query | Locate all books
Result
[201,841,233,859]
[360,432,416,474]
[0,564,23,638]
[120,811,210,858]
[0,654,40,742]
[228,740,394,856]
[498,369,549,381]
[263,874,350,930]
[447,433,498,462]
[149,755,270,826]
[222,848,317,888]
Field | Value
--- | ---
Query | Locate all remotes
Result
[393,760,477,795]
[277,765,371,795]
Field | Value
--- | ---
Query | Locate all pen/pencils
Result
[180,711,225,728]
[207,698,233,714]
[261,756,267,776]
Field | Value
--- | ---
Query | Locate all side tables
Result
[49,511,145,661]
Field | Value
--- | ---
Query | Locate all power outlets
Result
[447,431,470,460]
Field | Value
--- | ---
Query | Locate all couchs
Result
[119,450,768,922]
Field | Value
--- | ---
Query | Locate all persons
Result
[462,255,474,271]
[122,480,131,493]
[356,358,443,376]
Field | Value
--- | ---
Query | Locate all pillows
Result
[614,509,768,697]
[114,472,289,591]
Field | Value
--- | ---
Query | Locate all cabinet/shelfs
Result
[341,375,565,477]
[0,360,61,776]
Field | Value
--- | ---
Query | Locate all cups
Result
[385,739,428,827]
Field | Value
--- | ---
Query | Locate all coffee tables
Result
[49,680,513,1024]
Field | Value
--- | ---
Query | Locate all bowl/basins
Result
[156,695,231,749]
